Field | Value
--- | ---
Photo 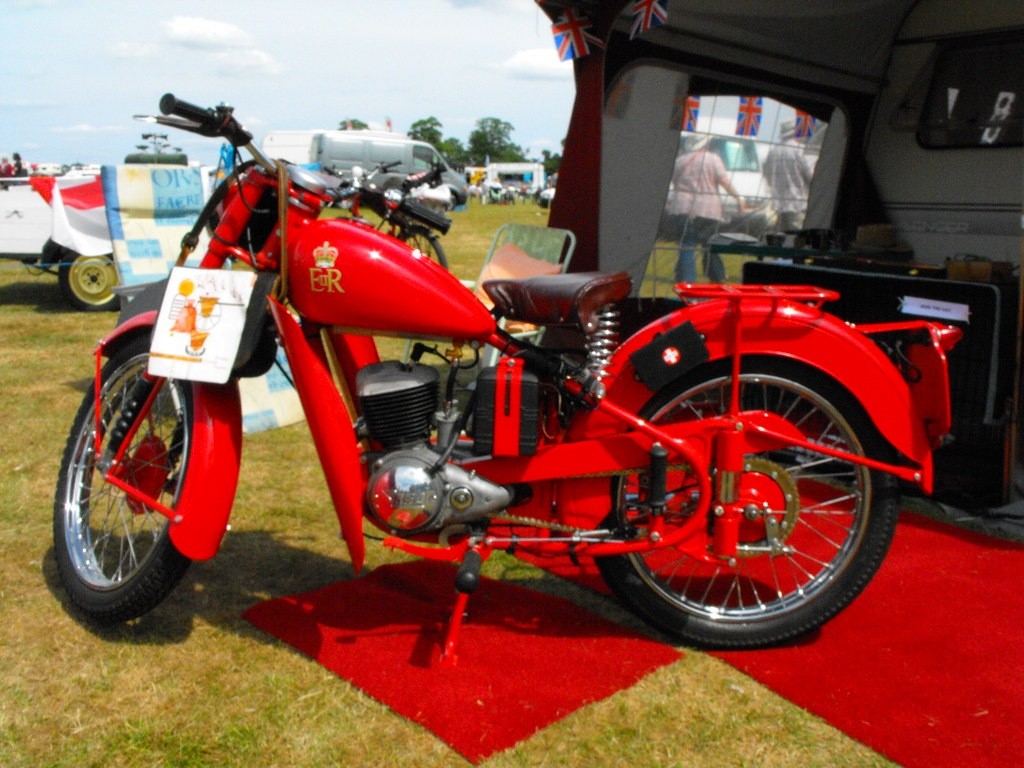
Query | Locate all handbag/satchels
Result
[172,157,290,378]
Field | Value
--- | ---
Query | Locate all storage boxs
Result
[948,261,1013,284]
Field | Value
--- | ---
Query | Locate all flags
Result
[603,69,816,138]
[629,0,669,41]
[551,6,606,63]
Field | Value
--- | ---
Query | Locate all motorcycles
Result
[47,91,960,652]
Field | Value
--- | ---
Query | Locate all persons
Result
[0,152,42,190]
[672,133,746,284]
[475,176,501,204]
[505,184,515,205]
[763,120,814,231]
[548,172,559,187]
[517,185,528,205]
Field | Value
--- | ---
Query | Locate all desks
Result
[742,261,1011,425]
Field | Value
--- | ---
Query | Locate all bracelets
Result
[738,196,745,200]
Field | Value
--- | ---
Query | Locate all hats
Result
[778,119,797,137]
[684,131,709,151]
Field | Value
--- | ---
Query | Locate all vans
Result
[660,131,776,231]
[305,132,469,212]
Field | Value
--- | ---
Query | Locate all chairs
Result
[403,225,574,368]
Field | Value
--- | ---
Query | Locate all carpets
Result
[490,439,1024,768]
[247,559,687,768]
